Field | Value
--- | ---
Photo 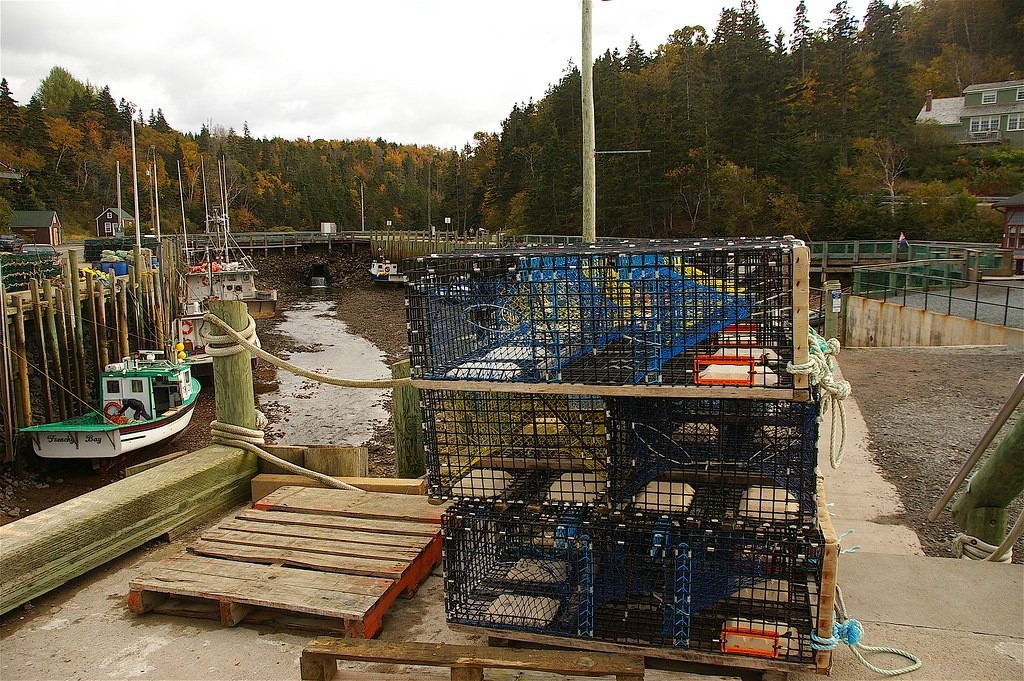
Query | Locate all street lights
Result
[354,175,365,232]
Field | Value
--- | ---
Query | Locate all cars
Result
[0,233,26,254]
[19,243,64,273]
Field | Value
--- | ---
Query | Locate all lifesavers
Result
[202,276,209,286]
[182,321,193,335]
[104,402,121,419]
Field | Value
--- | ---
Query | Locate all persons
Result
[117,398,152,420]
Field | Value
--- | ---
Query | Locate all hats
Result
[122,398,128,405]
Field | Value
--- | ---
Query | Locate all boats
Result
[17,337,202,462]
[366,251,408,284]
[159,154,279,384]
